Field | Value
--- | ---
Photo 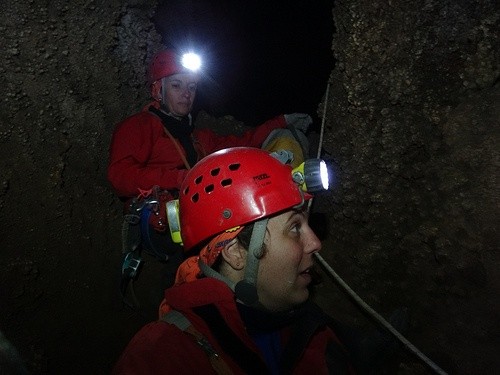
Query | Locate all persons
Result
[112,147,352,374]
[106,48,313,287]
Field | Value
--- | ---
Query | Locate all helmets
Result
[164,146,330,254]
[148,46,201,92]
